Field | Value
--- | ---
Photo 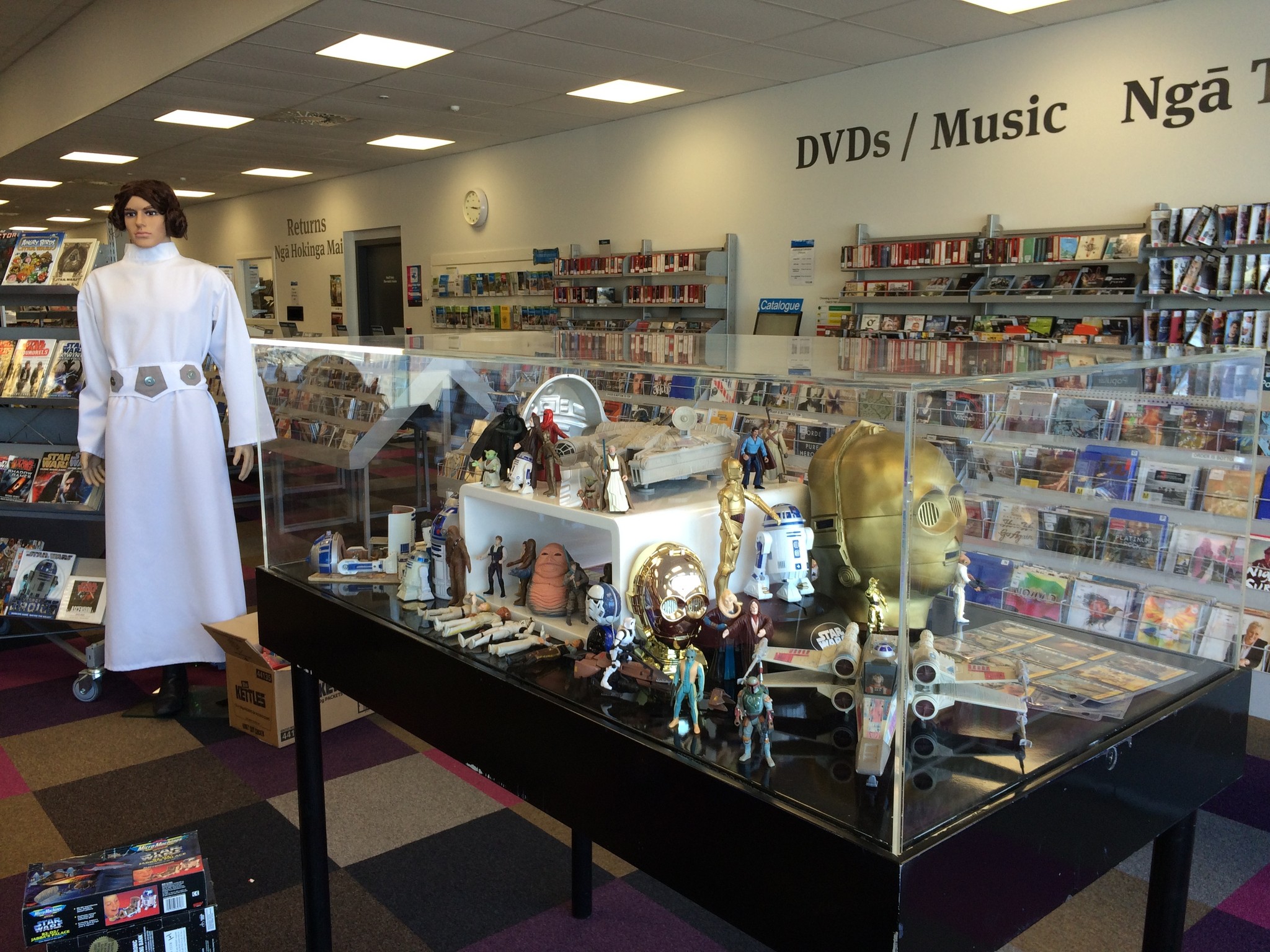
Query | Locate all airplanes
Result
[735,620,1032,785]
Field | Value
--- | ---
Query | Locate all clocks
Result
[462,188,489,228]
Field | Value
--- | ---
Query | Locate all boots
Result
[152,663,189,715]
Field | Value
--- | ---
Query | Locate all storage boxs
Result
[22,828,225,952]
[225,327,1269,952]
[202,608,390,748]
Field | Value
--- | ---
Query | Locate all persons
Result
[734,676,776,769]
[16,360,31,396]
[57,470,83,504]
[133,868,155,882]
[253,204,1270,680]
[103,894,124,920]
[668,648,705,734]
[29,361,44,397]
[74,178,275,716]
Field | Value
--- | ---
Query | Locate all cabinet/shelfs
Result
[834,200,1269,461]
[549,230,741,375]
